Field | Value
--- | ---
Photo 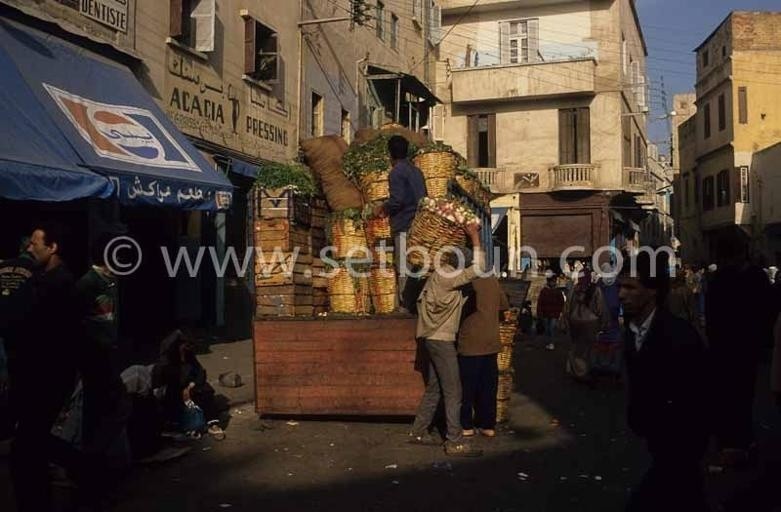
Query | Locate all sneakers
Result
[545,343,555,351]
[410,427,494,455]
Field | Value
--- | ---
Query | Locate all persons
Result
[454,248,513,438]
[535,223,780,511]
[373,132,429,312]
[0,223,228,512]
[405,220,485,457]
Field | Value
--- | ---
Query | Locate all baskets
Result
[327,150,494,316]
[496,321,517,422]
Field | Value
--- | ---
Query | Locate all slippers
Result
[207,424,225,441]
